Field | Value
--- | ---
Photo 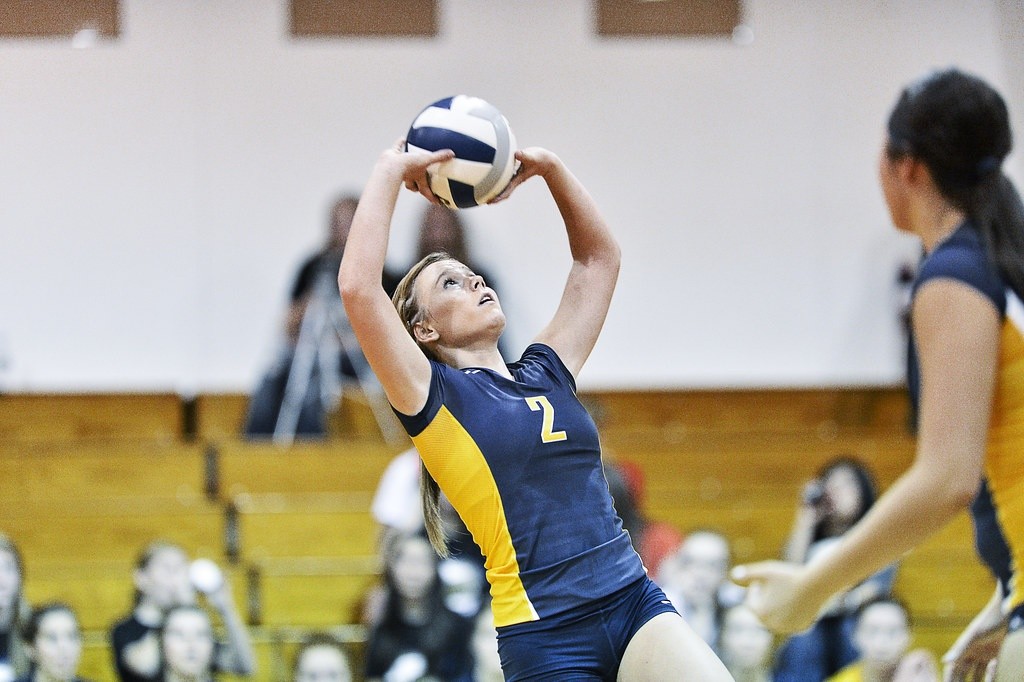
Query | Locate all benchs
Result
[0,387,1024,682]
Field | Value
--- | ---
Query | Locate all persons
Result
[0,443,505,682]
[338,143,734,682]
[730,70,1024,682]
[246,197,507,441]
[600,453,943,682]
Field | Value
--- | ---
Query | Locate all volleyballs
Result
[401,93,518,212]
